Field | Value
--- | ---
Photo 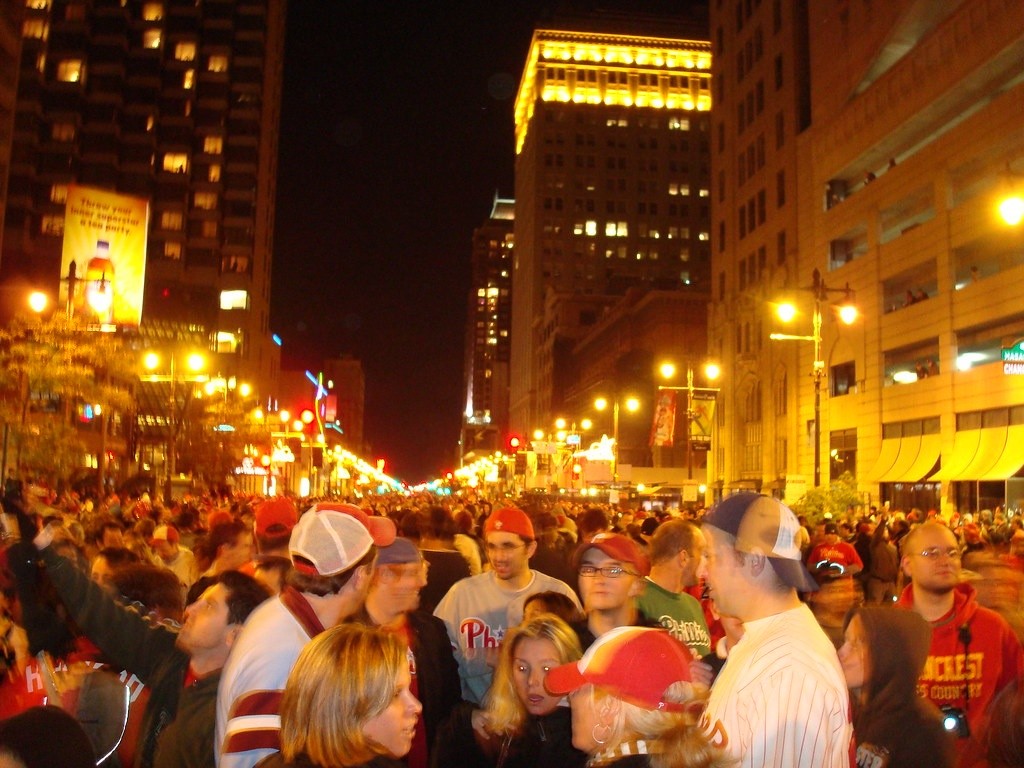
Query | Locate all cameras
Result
[939,704,970,738]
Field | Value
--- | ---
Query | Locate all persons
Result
[544,626,735,768]
[215,502,396,768]
[695,492,849,768]
[253,624,423,768]
[432,507,583,703]
[0,499,1024,768]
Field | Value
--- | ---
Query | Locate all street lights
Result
[594,396,642,485]
[658,357,730,477]
[137,327,207,502]
[27,259,112,495]
[781,266,864,491]
[203,371,339,498]
[494,417,593,493]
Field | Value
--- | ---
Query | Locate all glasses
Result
[907,548,961,561]
[485,543,528,551]
[576,567,641,579]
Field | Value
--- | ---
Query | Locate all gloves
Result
[41,555,75,591]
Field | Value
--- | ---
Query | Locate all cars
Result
[627,481,710,502]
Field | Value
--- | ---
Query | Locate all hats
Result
[148,525,179,544]
[572,532,651,577]
[208,512,234,528]
[372,536,421,565]
[288,502,397,576]
[254,495,299,538]
[824,524,840,535]
[702,493,821,592]
[487,509,536,540]
[543,626,706,713]
